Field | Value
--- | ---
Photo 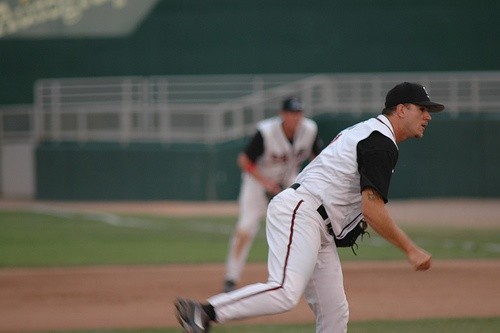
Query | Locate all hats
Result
[384,81,445,114]
[281,96,306,112]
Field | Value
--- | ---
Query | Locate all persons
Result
[222,96,326,292]
[172,82,445,333]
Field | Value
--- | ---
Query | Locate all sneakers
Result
[172,297,210,333]
[223,281,236,292]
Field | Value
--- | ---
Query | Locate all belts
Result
[290,182,337,240]
